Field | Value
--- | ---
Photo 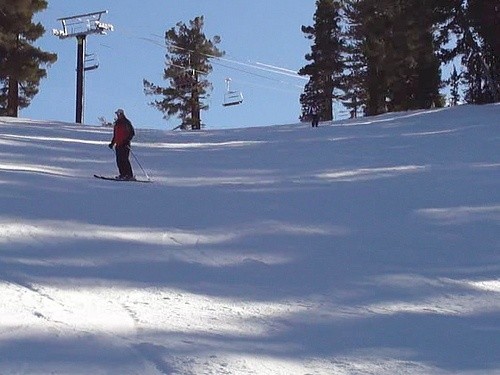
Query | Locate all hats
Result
[115,109,124,113]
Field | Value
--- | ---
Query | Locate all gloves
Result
[108,143,113,149]
[125,141,130,147]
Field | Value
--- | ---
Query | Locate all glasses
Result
[117,113,121,116]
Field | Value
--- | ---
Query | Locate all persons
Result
[108,109,135,179]
[308,101,322,127]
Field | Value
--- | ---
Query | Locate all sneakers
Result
[116,174,133,180]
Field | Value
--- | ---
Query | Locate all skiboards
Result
[93,174,153,183]
